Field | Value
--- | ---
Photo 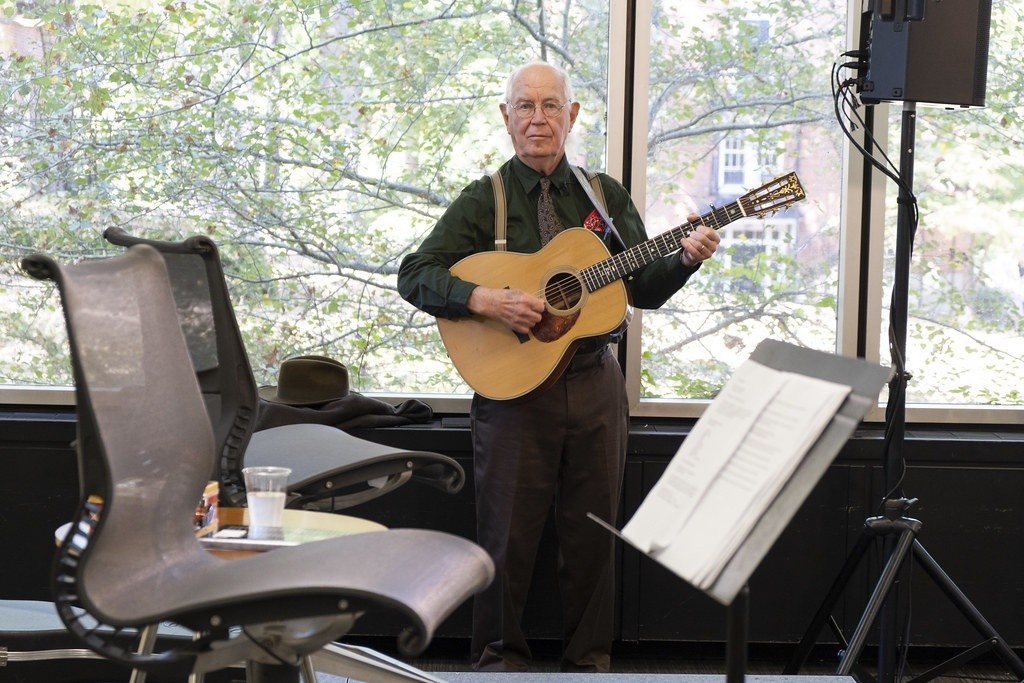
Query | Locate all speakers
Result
[857,0,993,107]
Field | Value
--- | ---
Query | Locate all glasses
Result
[507,100,571,118]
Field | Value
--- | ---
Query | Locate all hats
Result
[256,356,363,405]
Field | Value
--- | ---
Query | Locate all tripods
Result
[779,100,1024,683]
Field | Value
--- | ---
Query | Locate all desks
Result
[52,504,393,682]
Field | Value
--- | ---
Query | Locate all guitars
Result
[434,170,806,401]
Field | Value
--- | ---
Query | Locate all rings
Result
[700,245,704,251]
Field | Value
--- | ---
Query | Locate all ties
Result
[538,177,565,246]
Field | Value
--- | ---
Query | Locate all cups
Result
[243,466,292,527]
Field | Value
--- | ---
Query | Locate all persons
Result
[397,63,720,674]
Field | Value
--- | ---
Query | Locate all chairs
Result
[18,243,495,683]
[100,226,468,682]
